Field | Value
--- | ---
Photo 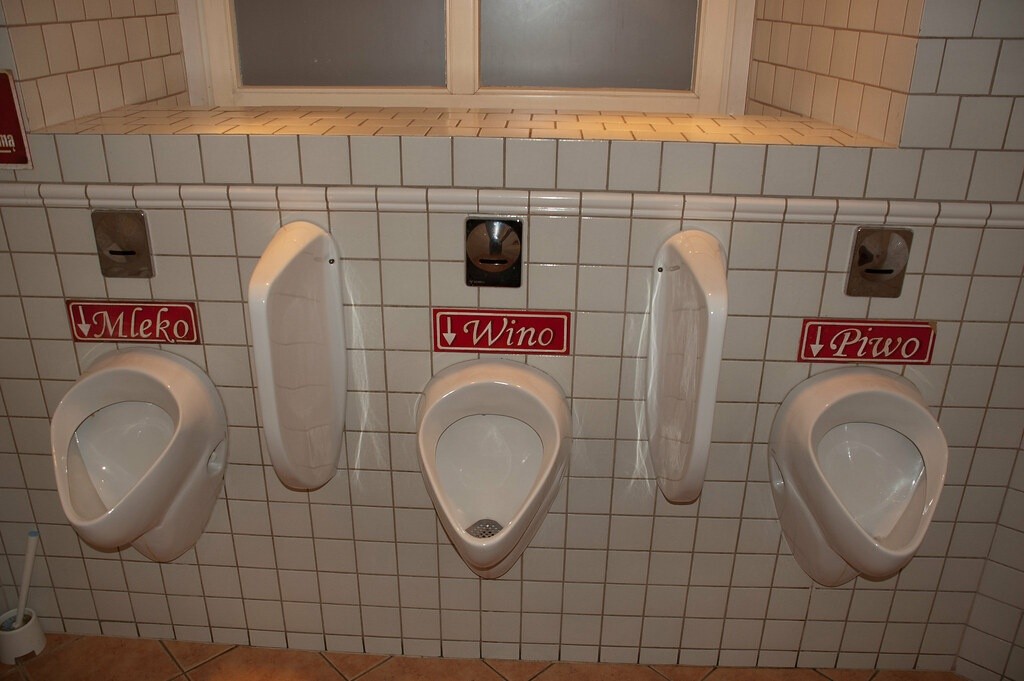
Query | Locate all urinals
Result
[49,347,229,565]
[415,358,574,580]
[768,365,949,585]
